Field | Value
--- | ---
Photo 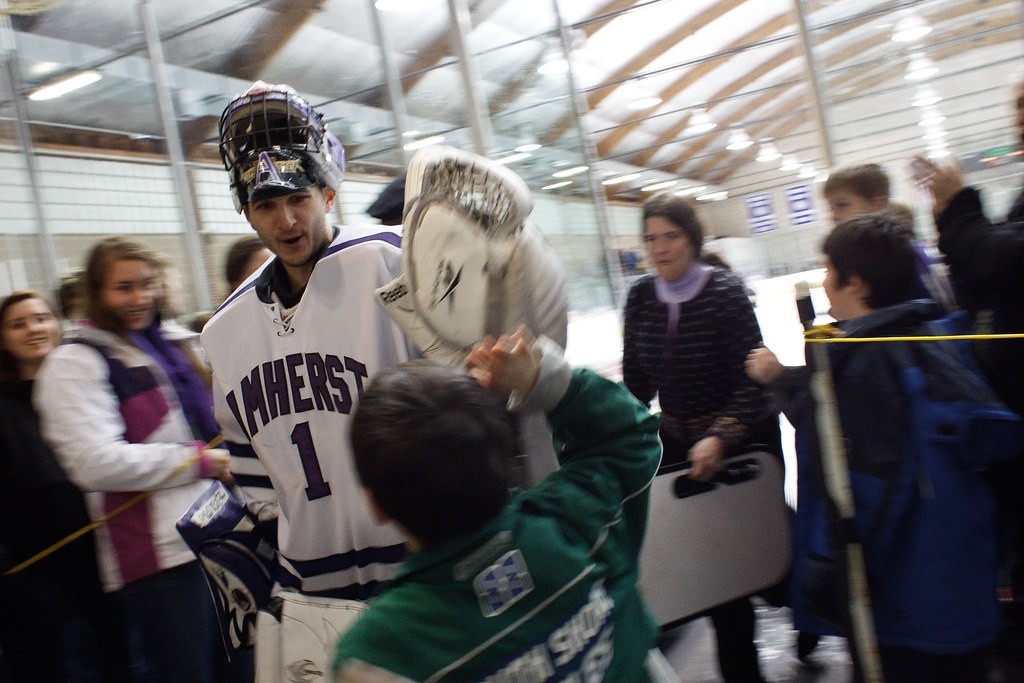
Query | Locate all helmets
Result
[220,79,346,215]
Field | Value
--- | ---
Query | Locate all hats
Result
[367,162,466,215]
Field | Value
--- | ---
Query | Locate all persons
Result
[329,325,663,683]
[744,78,1024,683]
[622,193,798,683]
[0,236,276,683]
[198,81,568,683]
